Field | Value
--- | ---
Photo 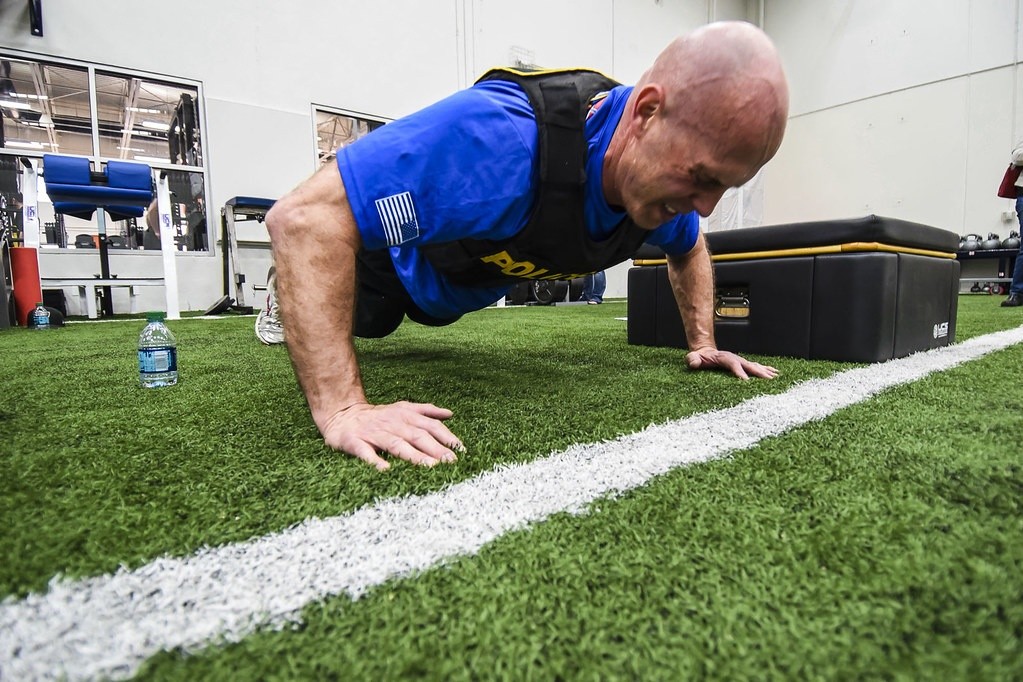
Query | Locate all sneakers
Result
[255,274,286,345]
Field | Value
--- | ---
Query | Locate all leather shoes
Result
[1001,295,1022,306]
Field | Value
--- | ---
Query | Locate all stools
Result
[627,214,962,364]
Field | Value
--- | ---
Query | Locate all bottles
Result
[139,314,177,387]
[34,302,50,329]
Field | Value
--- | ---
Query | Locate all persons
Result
[998,140,1023,307]
[255,21,788,471]
[144,191,208,251]
[12,194,24,248]
